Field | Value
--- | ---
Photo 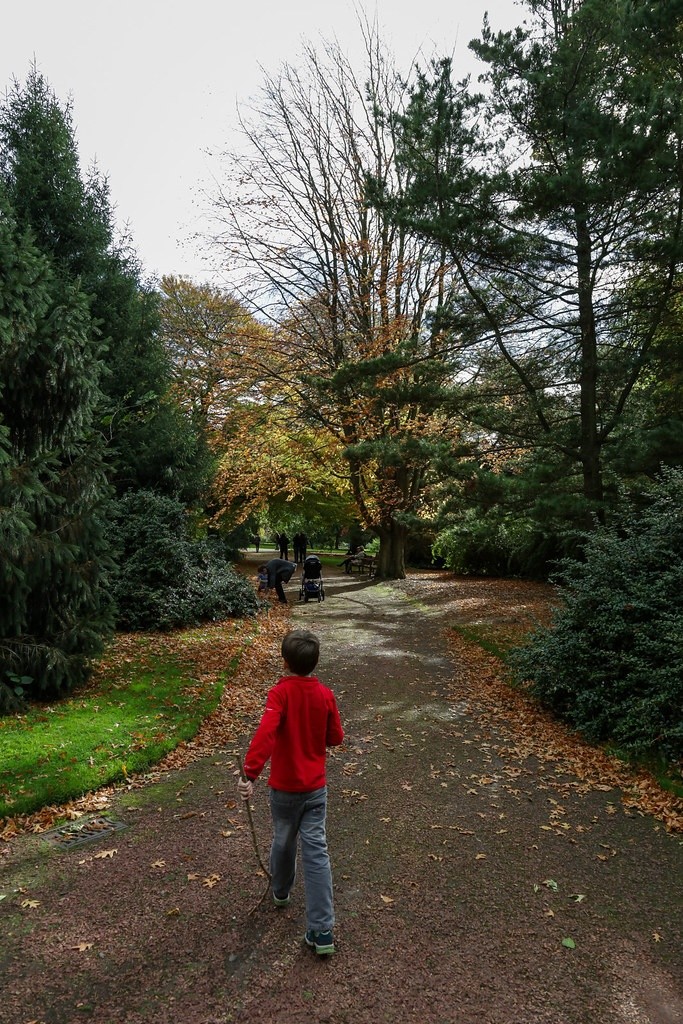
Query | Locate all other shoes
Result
[279,600,287,604]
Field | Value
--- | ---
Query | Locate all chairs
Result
[350,553,367,573]
[360,552,379,578]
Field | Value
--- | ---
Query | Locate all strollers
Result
[300,554,325,603]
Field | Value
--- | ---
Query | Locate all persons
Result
[256,572,270,594]
[258,558,298,605]
[237,628,346,956]
[254,530,309,565]
[335,546,366,576]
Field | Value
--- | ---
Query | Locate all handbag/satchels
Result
[301,547,306,553]
[306,581,318,590]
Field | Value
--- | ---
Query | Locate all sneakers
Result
[273,891,289,905]
[305,929,335,954]
